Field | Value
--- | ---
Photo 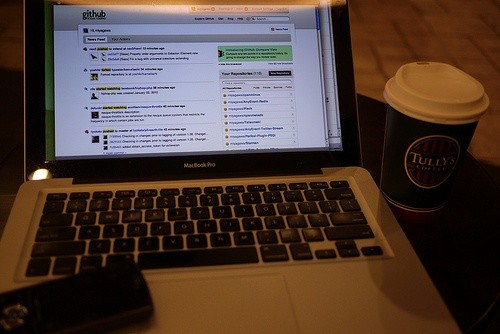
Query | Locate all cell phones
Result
[0,258,154,334]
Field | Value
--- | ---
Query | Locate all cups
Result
[379,61,490,213]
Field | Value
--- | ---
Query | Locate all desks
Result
[0,67,500,334]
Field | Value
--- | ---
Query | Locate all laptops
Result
[0,0,462,334]
